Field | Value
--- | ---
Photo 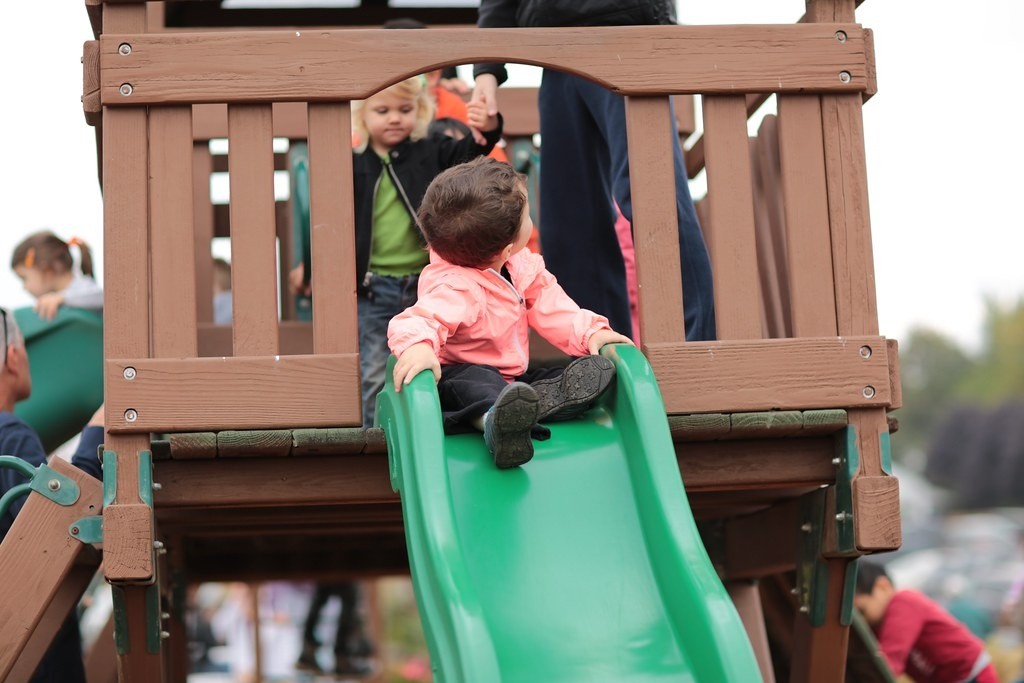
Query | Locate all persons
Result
[386,158,637,470]
[213,0,717,427]
[0,231,105,683]
[854,525,1023,683]
[184,578,376,677]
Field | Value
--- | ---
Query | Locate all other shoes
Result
[294,646,374,678]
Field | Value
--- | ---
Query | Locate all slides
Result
[373,340,774,683]
[3,299,105,453]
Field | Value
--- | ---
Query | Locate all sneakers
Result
[536,353,618,422]
[483,381,541,471]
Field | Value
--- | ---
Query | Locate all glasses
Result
[0,303,11,372]
